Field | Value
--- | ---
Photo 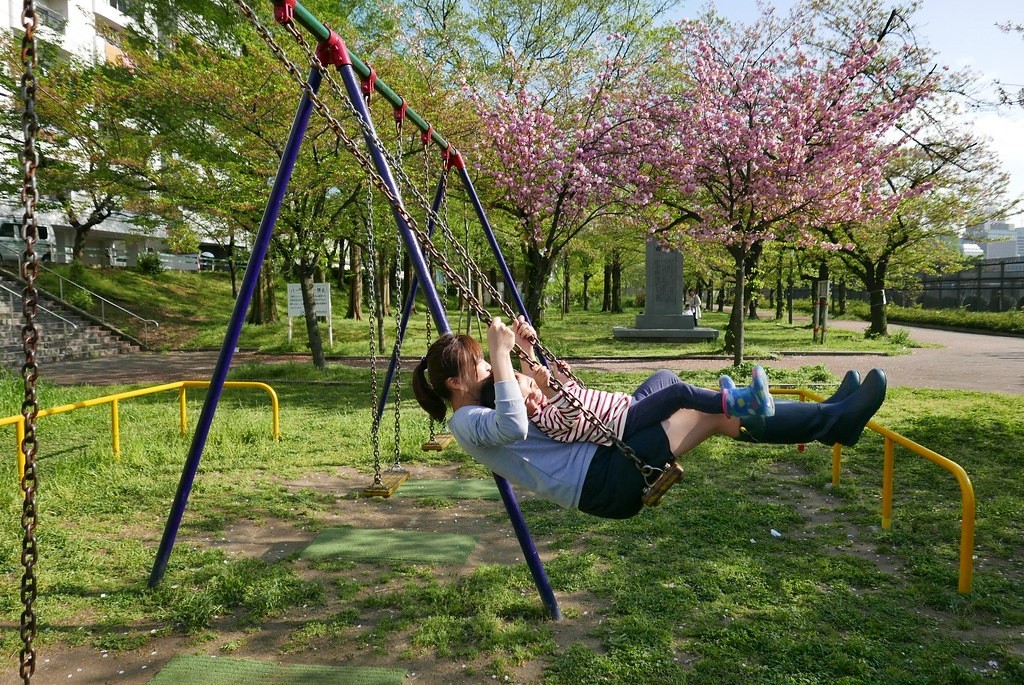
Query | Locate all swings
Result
[233,0,683,507]
[419,132,455,453]
[359,79,409,499]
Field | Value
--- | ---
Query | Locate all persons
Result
[481,360,776,446]
[412,333,887,519]
[689,289,702,327]
[682,304,693,315]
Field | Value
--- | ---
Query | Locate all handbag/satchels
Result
[694,294,702,306]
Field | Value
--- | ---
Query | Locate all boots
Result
[816,370,861,447]
[718,374,738,393]
[722,364,774,420]
[731,368,885,445]
